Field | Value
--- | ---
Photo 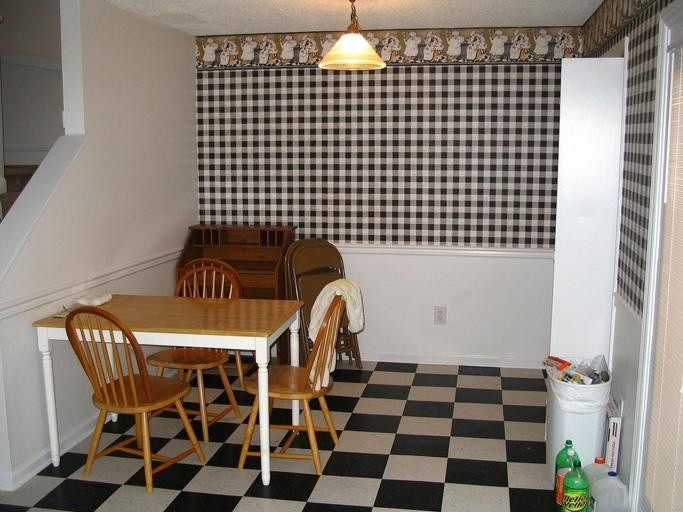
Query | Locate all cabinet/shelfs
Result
[176,225,298,365]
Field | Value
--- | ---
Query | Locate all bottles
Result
[553,439,581,495]
[560,461,590,512]
[554,449,577,511]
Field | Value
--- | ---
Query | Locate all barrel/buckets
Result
[592,471,630,512]
[584,456,613,487]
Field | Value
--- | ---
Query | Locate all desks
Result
[30,294,304,487]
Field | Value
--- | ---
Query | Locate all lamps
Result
[318,1,387,72]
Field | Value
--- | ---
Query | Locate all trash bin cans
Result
[545,357,612,487]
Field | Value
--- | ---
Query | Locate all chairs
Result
[145,258,242,445]
[178,258,245,391]
[237,293,346,476]
[64,305,205,494]
[284,238,362,370]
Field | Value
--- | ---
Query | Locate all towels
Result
[307,278,366,392]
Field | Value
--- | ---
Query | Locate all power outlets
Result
[434,305,448,326]
